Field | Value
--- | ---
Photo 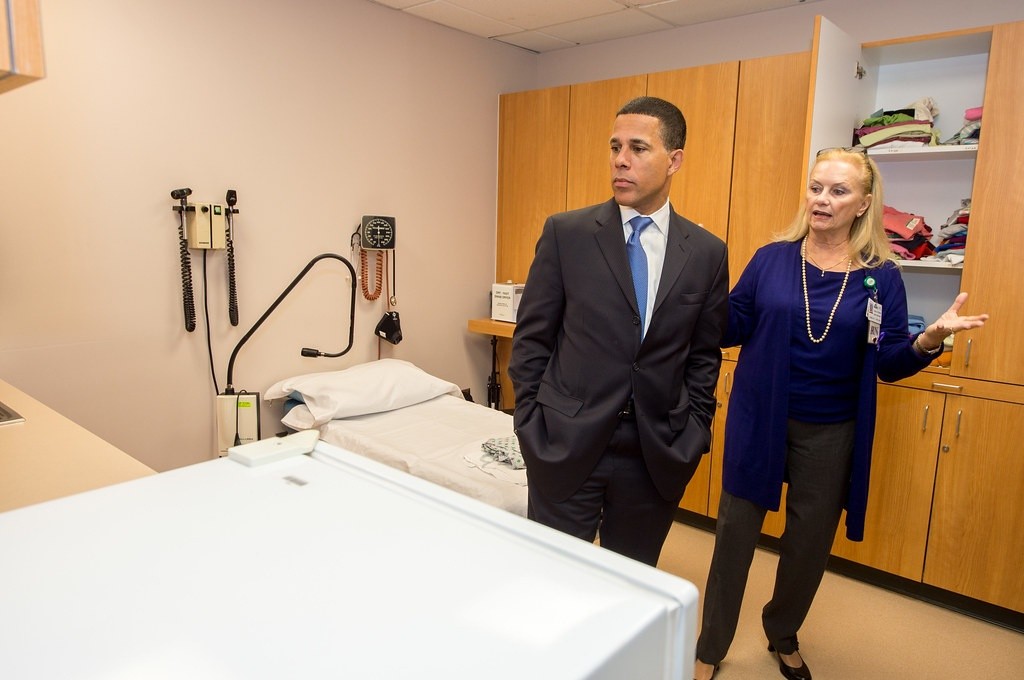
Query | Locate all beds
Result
[263,358,528,518]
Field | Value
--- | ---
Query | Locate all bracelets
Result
[916,332,941,356]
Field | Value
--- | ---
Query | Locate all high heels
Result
[692,663,719,680]
[767,638,811,680]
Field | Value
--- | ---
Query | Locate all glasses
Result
[816,146,873,196]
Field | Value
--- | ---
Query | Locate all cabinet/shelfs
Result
[495,16,1024,617]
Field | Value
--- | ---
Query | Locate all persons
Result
[694,148,988,680]
[507,97,729,569]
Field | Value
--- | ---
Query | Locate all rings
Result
[949,326,955,334]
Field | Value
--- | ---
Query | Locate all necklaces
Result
[805,250,849,277]
[802,234,852,344]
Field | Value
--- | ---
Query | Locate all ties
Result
[627,216,654,341]
